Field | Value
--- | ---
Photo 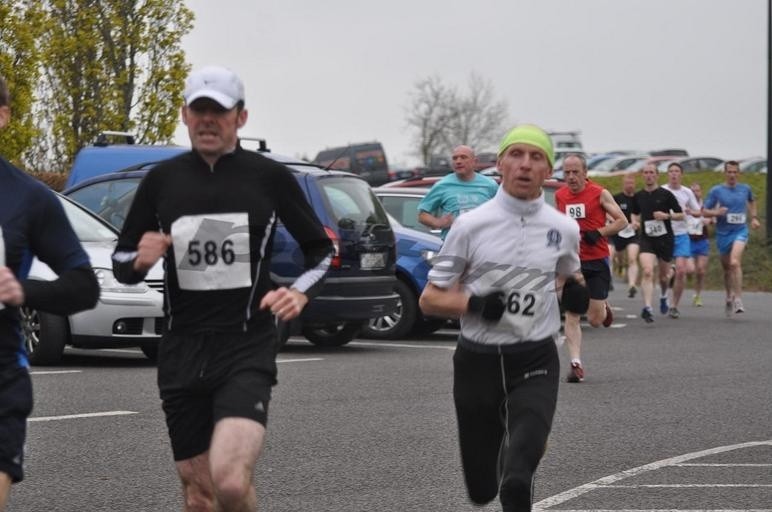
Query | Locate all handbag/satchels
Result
[749,216,758,220]
[667,214,671,220]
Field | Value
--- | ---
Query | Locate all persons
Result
[659,164,701,318]
[555,151,628,383]
[702,160,760,319]
[631,163,685,323]
[111,66,333,511]
[687,184,718,308]
[607,174,643,297]
[417,144,502,245]
[415,124,591,512]
[0,76,102,510]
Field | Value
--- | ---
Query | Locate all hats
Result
[541,129,767,185]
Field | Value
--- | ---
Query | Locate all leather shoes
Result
[583,228,602,246]
[467,290,507,320]
[562,275,590,314]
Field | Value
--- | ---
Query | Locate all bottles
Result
[497,123,554,166]
[183,66,247,110]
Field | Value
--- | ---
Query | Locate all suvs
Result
[56,136,402,348]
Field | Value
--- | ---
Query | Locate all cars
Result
[541,129,767,185]
[357,164,499,341]
[14,189,166,365]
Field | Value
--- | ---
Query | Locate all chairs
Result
[567,361,585,383]
[686,274,694,287]
[627,285,638,298]
[669,263,676,288]
[660,294,669,314]
[668,307,680,319]
[693,295,703,307]
[724,292,745,319]
[602,299,613,327]
[641,306,655,323]
